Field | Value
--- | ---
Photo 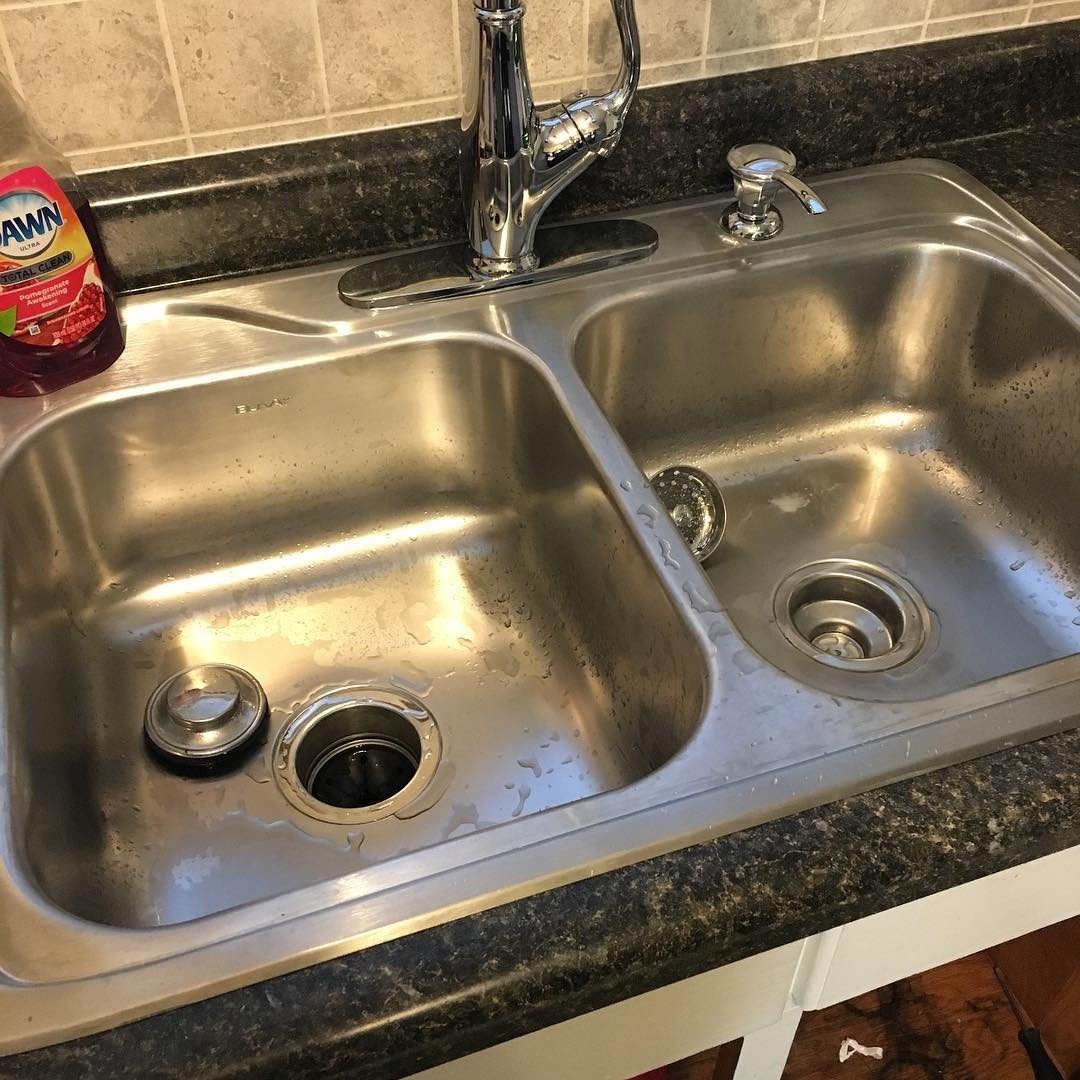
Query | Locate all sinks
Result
[492,147,1077,798]
[0,252,750,1056]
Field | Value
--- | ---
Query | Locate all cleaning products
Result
[0,53,127,408]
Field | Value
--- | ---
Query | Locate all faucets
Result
[458,1,662,291]
[717,143,829,245]
